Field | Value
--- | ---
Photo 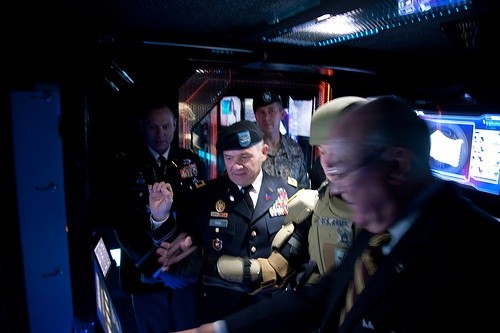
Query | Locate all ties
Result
[242,185,254,216]
[157,156,167,175]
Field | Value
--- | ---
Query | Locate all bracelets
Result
[242,256,252,285]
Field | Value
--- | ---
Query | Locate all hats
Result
[253,89,281,114]
[216,120,263,150]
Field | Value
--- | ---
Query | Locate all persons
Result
[111,89,500,333]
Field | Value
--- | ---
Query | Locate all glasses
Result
[328,143,403,193]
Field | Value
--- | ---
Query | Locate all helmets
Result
[309,96,369,145]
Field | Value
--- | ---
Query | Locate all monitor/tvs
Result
[89,248,124,333]
[422,112,500,203]
